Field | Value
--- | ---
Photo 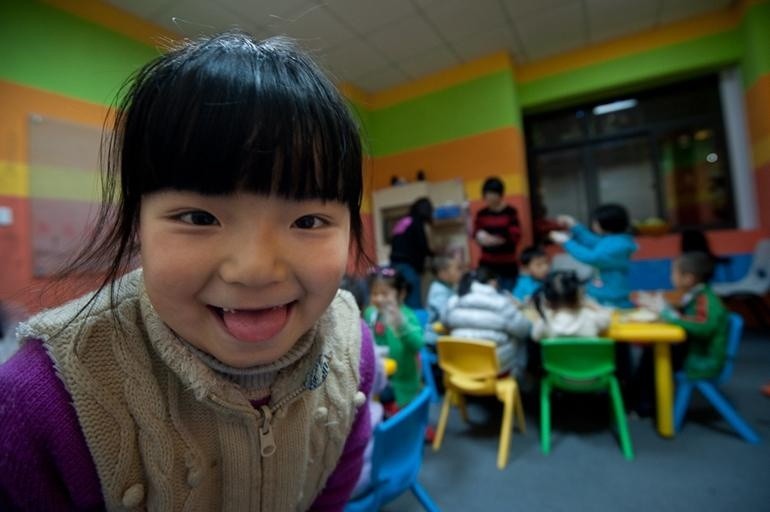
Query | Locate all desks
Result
[417,314,683,437]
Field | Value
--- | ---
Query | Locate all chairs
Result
[538,336,639,463]
[672,312,759,445]
[345,387,445,510]
[430,339,527,468]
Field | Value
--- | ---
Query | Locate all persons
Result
[676,226,730,285]
[426,250,461,330]
[551,202,636,418]
[529,269,612,341]
[441,266,533,386]
[0,30,378,512]
[362,265,417,421]
[623,253,728,417]
[389,193,436,308]
[468,175,521,273]
[514,247,551,300]
[334,272,399,500]
